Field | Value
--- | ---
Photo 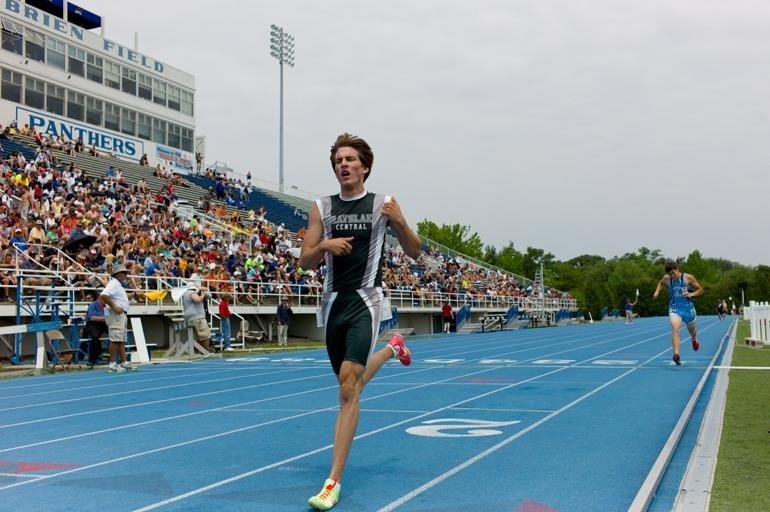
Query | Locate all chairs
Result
[45,329,83,374]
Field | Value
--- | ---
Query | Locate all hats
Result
[110,265,131,276]
[35,219,44,224]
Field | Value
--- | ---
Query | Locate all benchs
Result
[0,135,211,211]
[211,165,316,242]
[387,227,456,276]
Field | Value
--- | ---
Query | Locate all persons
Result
[381,243,441,307]
[243,207,327,306]
[717,299,745,320]
[436,257,572,308]
[86,294,109,367]
[0,145,260,305]
[277,296,293,346]
[100,264,137,372]
[442,301,454,333]
[622,298,637,325]
[182,284,211,352]
[653,264,704,365]
[299,131,422,511]
[219,293,234,350]
[1,119,99,157]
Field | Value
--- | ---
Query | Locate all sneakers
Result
[107,362,138,373]
[308,485,340,510]
[673,354,681,366]
[692,337,699,351]
[389,334,411,366]
